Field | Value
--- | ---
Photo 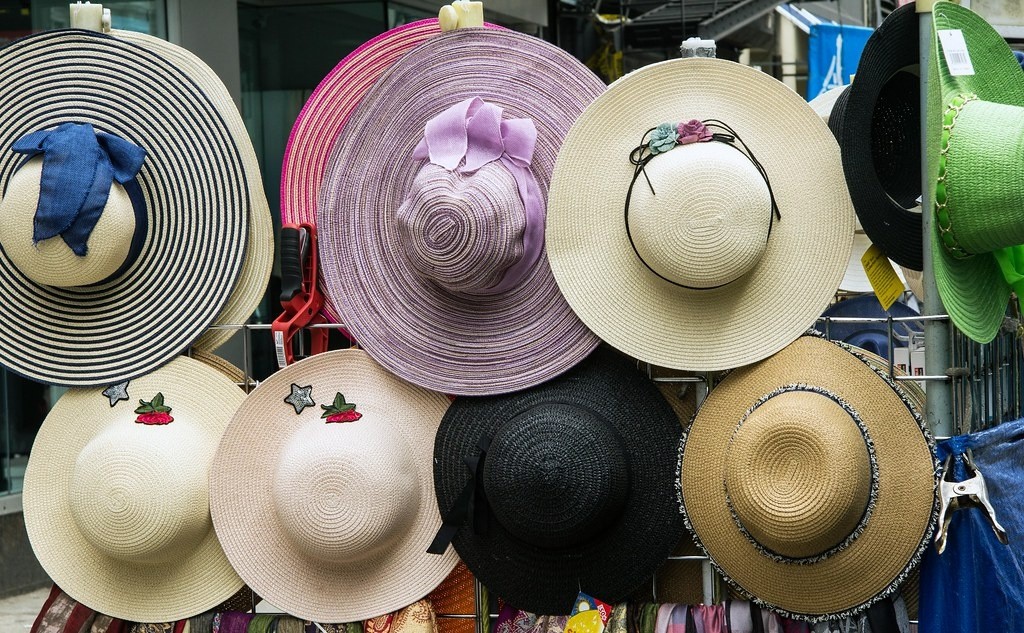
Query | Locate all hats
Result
[281,17,610,395]
[545,55,856,373]
[0,24,274,388]
[206,349,464,624]
[21,343,266,628]
[807,0,1024,348]
[423,339,943,624]
